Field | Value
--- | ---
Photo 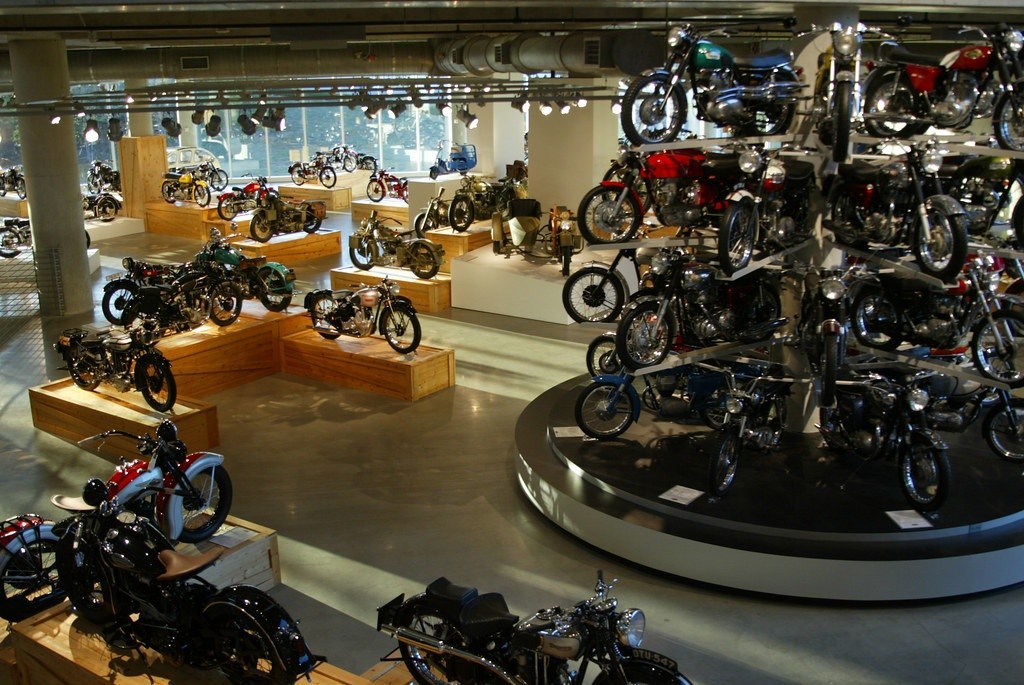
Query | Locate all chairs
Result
[507,198,542,218]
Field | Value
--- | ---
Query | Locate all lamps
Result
[50,84,622,142]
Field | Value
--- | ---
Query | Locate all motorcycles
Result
[348,211,446,279]
[304,274,422,354]
[81,159,123,222]
[782,15,912,163]
[51,477,328,685]
[562,218,797,497]
[0,418,232,622]
[162,143,410,243]
[774,252,1024,406]
[0,164,91,259]
[938,139,1024,248]
[620,23,813,146]
[862,23,1024,152]
[52,306,177,412]
[577,126,819,274]
[376,569,695,685]
[414,140,585,277]
[102,222,302,337]
[822,137,968,284]
[815,348,1024,512]
